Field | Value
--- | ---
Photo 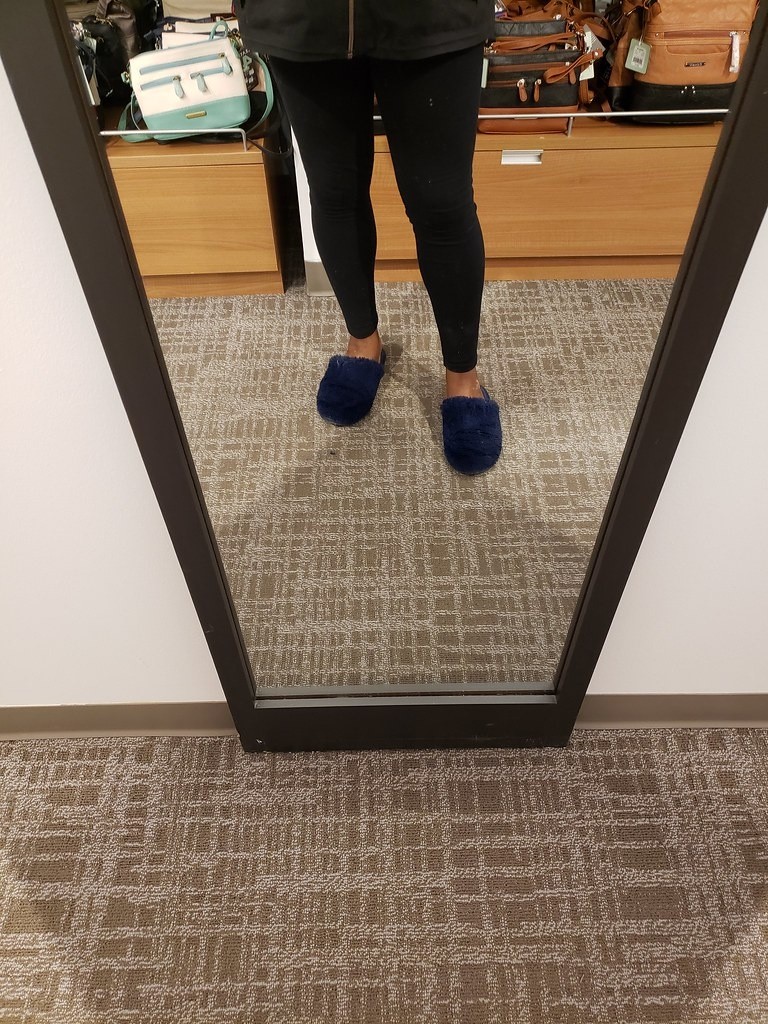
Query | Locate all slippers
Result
[441,386,502,476]
[316,347,386,427]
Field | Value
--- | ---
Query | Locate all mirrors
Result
[0,0,768,753]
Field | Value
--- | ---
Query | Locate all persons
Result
[233,0,504,474]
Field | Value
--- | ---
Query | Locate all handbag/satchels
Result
[66,0,272,145]
[477,0,761,134]
